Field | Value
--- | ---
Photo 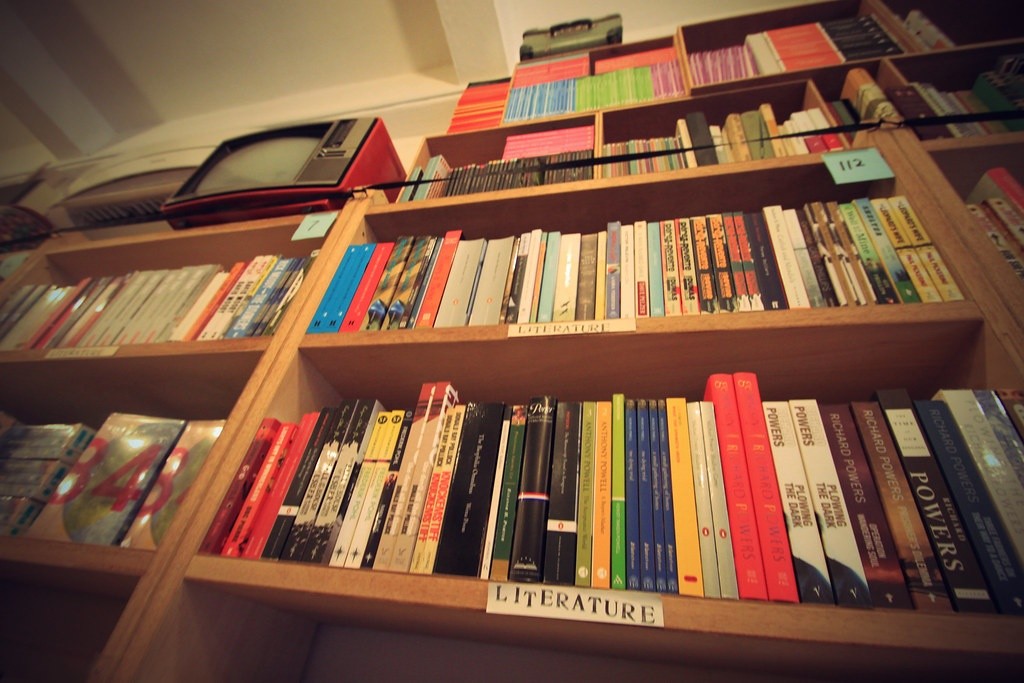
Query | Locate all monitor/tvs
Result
[162,115,406,227]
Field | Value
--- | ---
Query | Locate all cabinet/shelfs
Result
[0,0,1024,683]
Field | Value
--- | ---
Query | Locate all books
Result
[0,246,327,358]
[0,412,227,553]
[197,371,1024,617]
[397,47,1024,202]
[305,164,1024,334]
[687,10,957,87]
